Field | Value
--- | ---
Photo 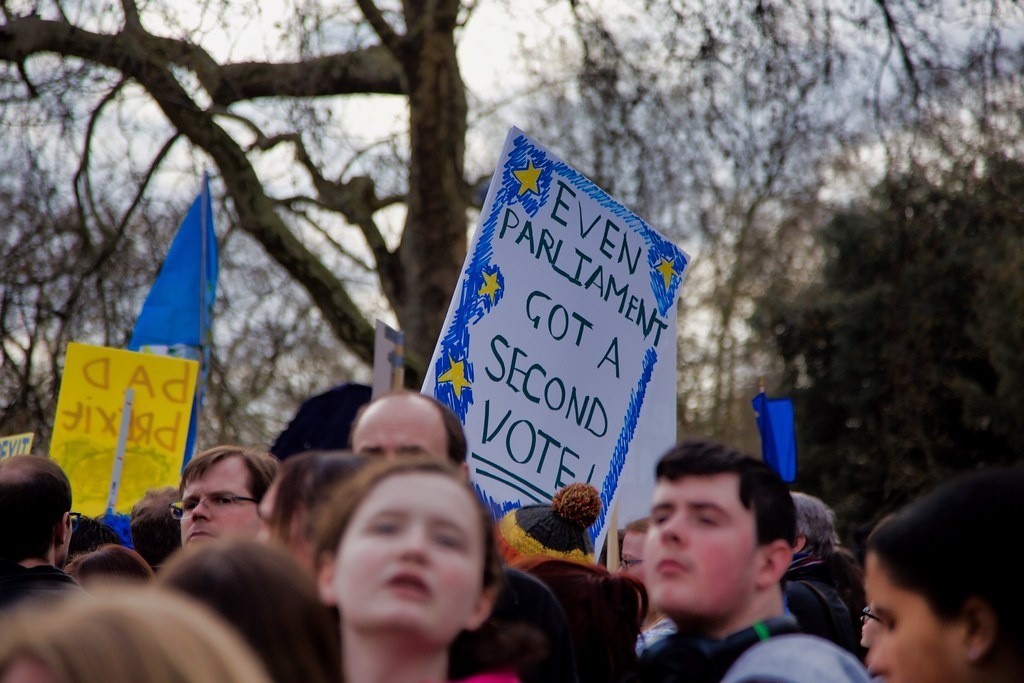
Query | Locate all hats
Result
[492,483,602,578]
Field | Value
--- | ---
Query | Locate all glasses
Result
[68,513,81,532]
[169,496,260,520]
[861,606,886,625]
[618,559,643,572]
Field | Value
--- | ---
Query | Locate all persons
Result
[644,440,872,683]
[317,393,678,683]
[862,470,1024,683]
[0,448,373,683]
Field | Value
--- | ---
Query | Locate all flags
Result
[752,392,796,481]
[127,171,219,472]
